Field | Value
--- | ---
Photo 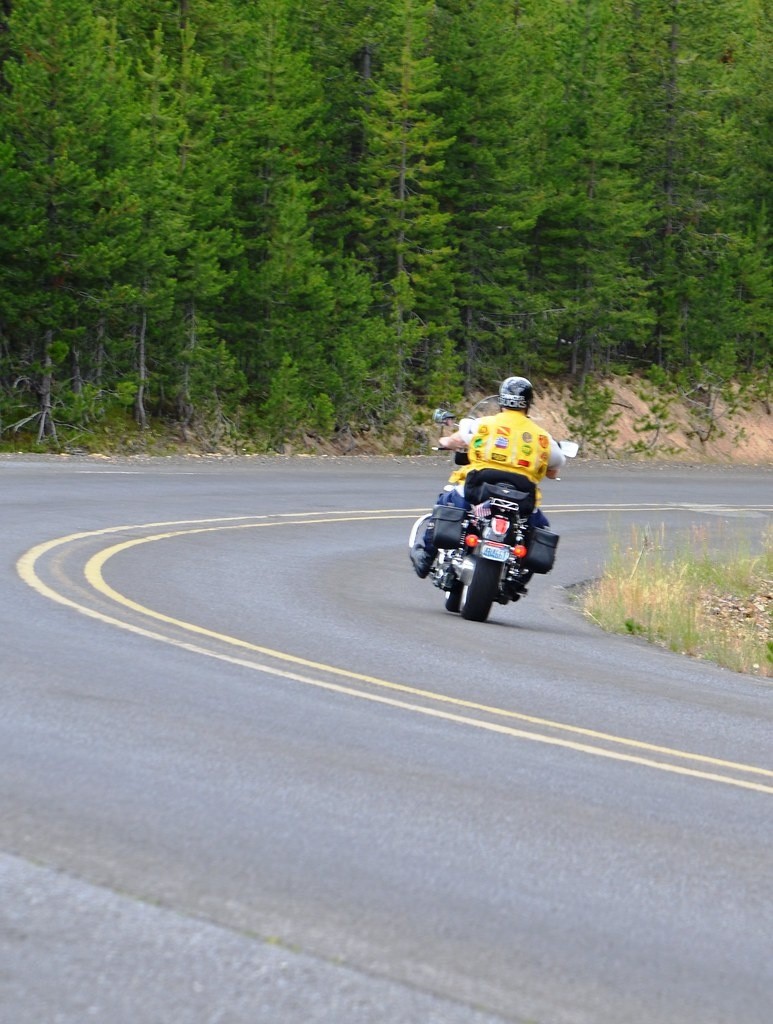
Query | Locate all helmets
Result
[498,376,533,409]
[442,412,456,420]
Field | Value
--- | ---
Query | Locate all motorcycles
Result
[408,395,579,621]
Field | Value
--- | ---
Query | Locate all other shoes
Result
[410,544,436,579]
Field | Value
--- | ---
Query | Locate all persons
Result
[441,412,455,426]
[407,374,567,592]
